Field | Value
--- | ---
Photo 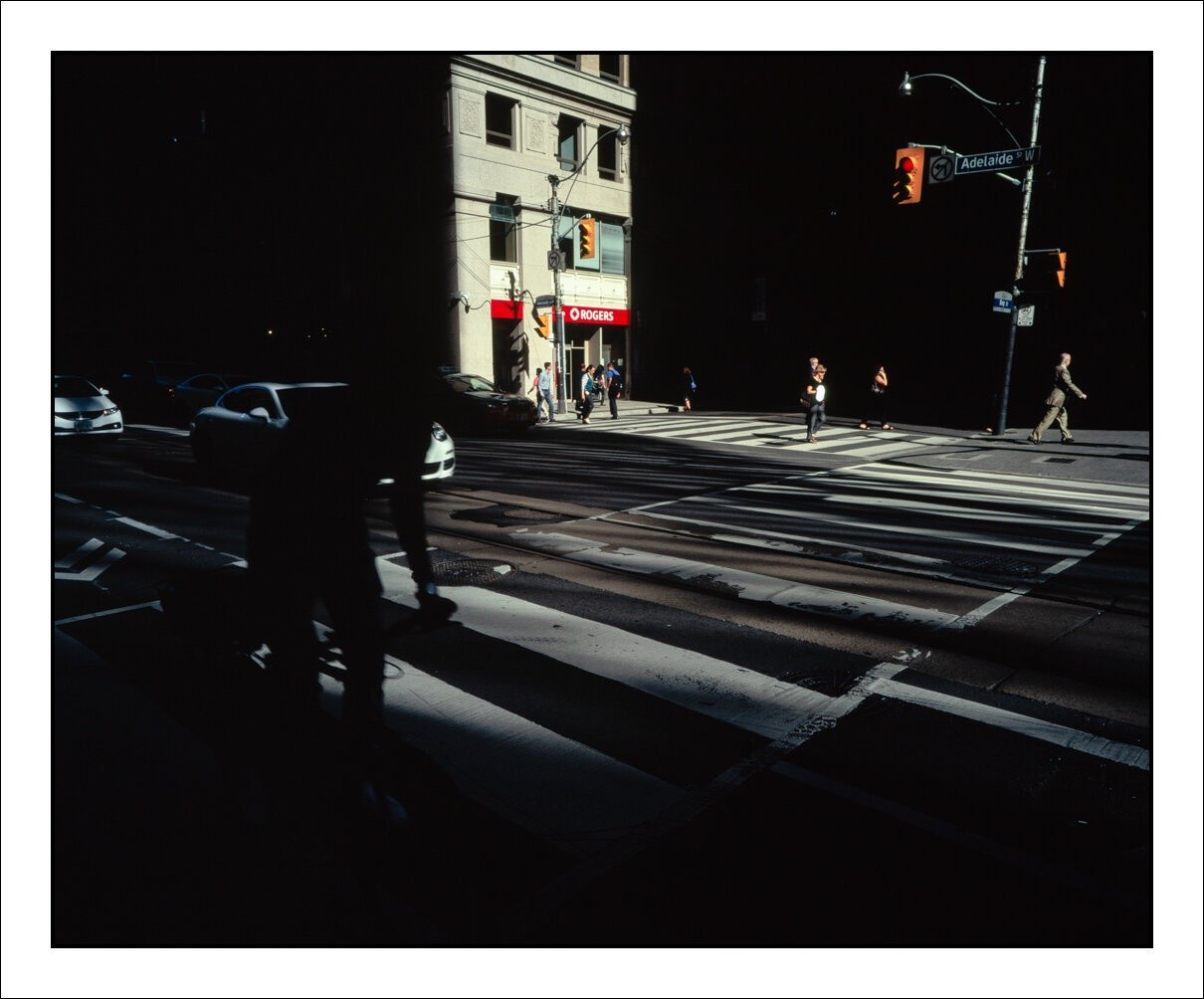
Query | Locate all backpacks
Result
[610,370,622,395]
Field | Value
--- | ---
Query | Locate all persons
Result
[800,357,827,443]
[1025,352,1086,445]
[575,362,623,423]
[535,362,556,423]
[683,367,696,414]
[858,364,895,430]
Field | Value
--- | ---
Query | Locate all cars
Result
[430,372,539,438]
[166,372,245,420]
[53,375,124,443]
[187,382,455,496]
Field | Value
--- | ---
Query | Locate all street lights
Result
[897,55,1047,436]
[547,121,628,413]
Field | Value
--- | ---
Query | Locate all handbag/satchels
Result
[871,382,885,395]
[691,374,696,389]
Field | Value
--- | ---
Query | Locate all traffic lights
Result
[893,145,925,205]
[578,218,595,259]
[1047,251,1068,291]
[535,315,549,338]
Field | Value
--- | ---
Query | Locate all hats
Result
[813,365,827,374]
